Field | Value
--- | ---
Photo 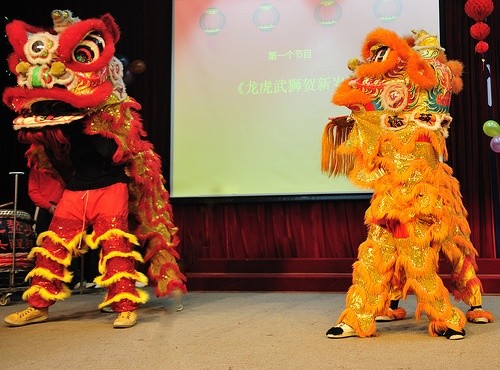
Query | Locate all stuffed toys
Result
[0,11,185,329]
[321,27,494,340]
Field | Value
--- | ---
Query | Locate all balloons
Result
[119,57,146,87]
[483,119,500,152]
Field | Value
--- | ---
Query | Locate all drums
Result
[0,209,38,272]
